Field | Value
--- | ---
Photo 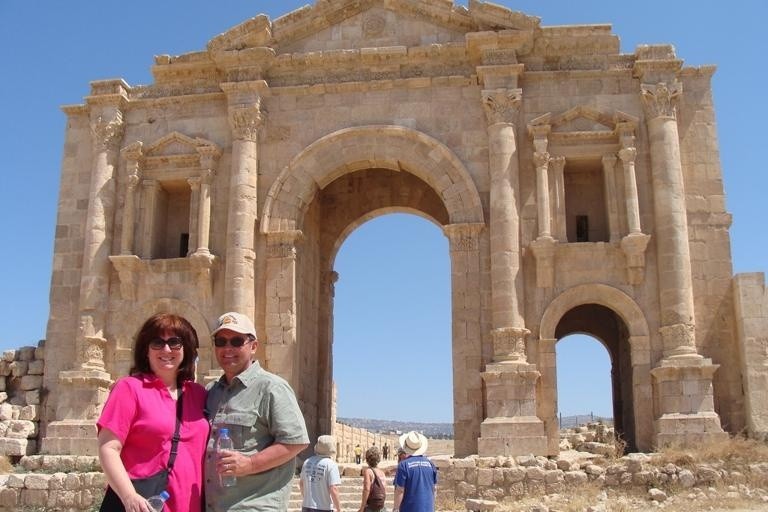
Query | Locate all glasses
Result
[212,336,253,348]
[147,336,184,351]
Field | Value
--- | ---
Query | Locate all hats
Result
[313,434,337,456]
[208,312,258,341]
[398,430,428,457]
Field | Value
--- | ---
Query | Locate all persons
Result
[206,312,311,511]
[357,447,389,511]
[299,434,344,511]
[353,443,362,464]
[382,442,389,461]
[391,431,438,511]
[94,312,207,511]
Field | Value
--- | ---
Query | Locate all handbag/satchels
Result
[366,467,386,511]
[96,469,170,511]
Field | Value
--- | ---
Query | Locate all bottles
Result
[216,429,237,488]
[146,491,170,512]
